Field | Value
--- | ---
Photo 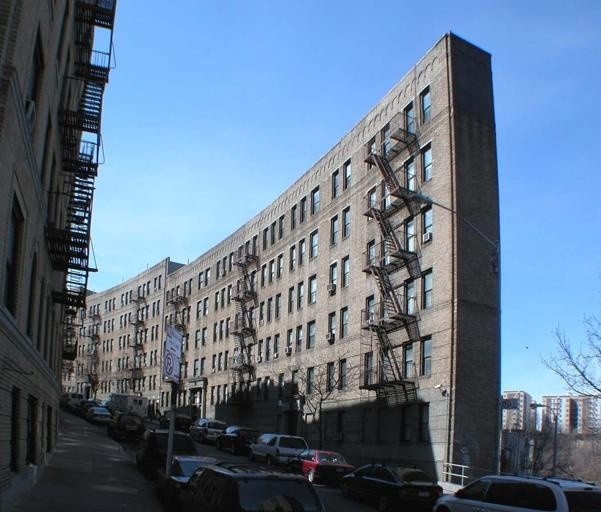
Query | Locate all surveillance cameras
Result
[434,383,443,389]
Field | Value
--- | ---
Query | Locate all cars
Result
[155,455,222,498]
[286,449,356,484]
[215,426,264,455]
[160,411,192,430]
[190,419,229,443]
[136,429,199,472]
[340,462,443,511]
[61,392,112,424]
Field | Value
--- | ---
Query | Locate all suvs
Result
[107,409,145,441]
[431,475,601,512]
[177,462,327,512]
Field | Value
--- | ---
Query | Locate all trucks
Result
[105,393,149,418]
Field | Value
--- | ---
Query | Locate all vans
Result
[247,434,310,466]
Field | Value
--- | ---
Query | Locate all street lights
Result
[162,324,187,506]
[530,403,558,478]
